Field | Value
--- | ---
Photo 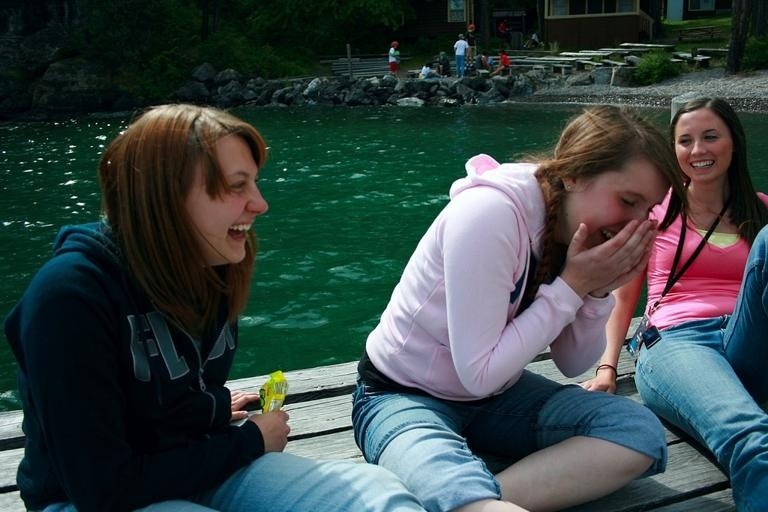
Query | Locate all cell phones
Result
[642,326,661,349]
[626,313,650,356]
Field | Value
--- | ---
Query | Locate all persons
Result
[1,98,430,512]
[415,22,546,79]
[566,89,768,509]
[388,40,401,78]
[341,102,686,510]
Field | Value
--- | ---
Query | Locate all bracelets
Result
[592,362,618,379]
